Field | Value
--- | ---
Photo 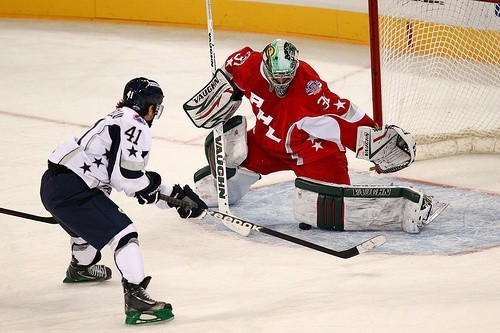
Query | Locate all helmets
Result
[123,77,163,116]
[262,40,299,98]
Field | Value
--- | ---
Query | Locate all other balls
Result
[298,222,312,231]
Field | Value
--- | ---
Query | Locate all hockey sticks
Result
[159,193,388,259]
[206,0,250,237]
[0,206,59,224]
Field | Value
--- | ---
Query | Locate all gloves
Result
[168,183,208,220]
[134,170,163,204]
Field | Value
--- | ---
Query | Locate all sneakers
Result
[62,249,112,283]
[121,274,174,325]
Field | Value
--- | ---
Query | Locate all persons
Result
[183,39,450,234]
[40,77,208,316]
